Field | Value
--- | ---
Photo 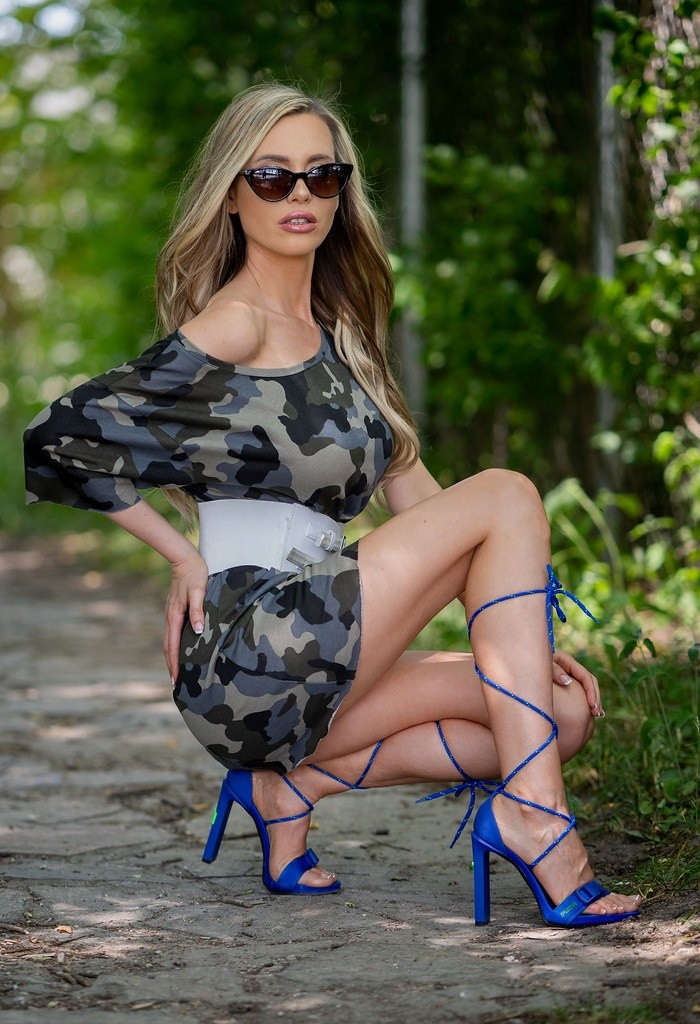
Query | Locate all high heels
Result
[203,718,501,896]
[469,563,642,928]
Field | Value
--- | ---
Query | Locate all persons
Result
[21,87,648,927]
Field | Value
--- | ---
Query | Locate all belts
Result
[196,499,348,575]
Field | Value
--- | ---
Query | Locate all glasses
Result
[237,162,354,202]
